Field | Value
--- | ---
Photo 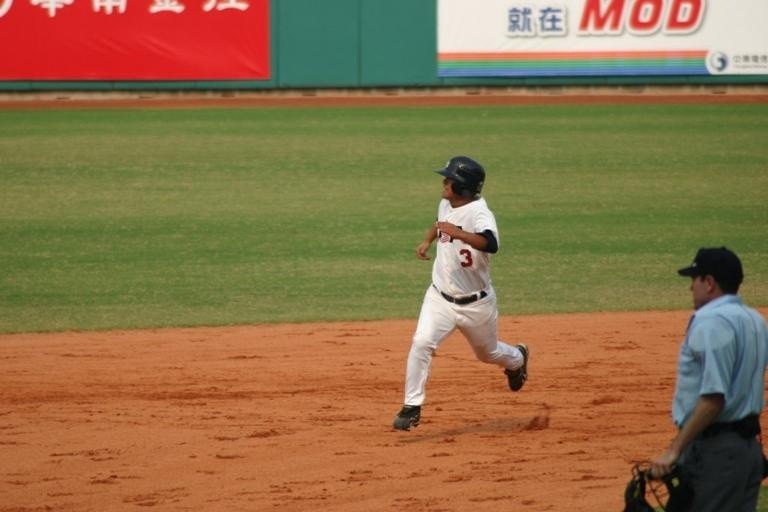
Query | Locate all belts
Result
[431,282,488,306]
[696,419,739,439]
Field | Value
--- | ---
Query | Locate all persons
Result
[646,245,768,512]
[392,156,528,431]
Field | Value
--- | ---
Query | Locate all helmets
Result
[433,155,487,194]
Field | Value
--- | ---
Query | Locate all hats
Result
[676,244,746,296]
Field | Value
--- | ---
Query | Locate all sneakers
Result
[503,341,530,392]
[392,404,422,430]
